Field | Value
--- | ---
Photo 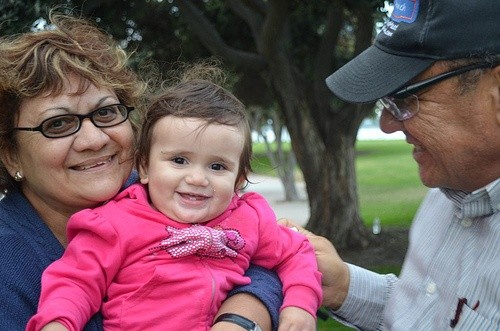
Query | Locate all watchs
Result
[213,312,263,331]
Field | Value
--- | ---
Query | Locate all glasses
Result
[375,59,500,122]
[7,100,136,140]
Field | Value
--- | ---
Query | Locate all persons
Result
[274,0,500,330]
[26,66,324,331]
[0,7,284,331]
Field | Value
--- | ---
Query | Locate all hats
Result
[325,0,500,104]
